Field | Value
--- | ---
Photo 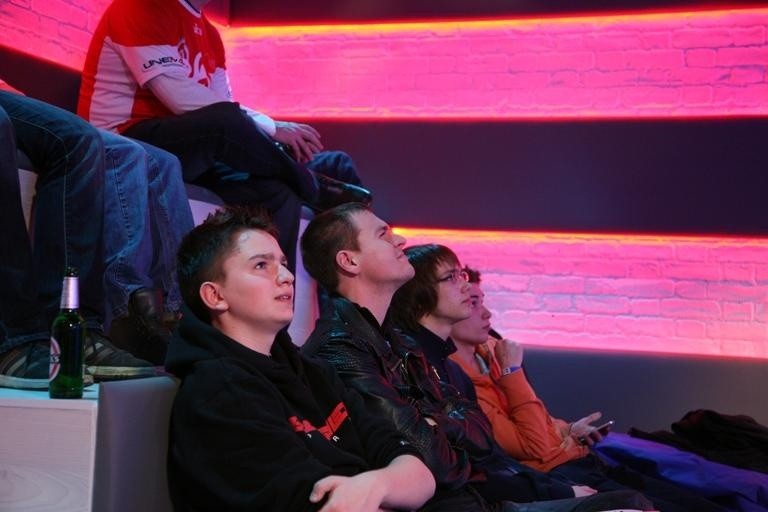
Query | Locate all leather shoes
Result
[301,170,373,215]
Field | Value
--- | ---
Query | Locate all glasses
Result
[432,268,471,285]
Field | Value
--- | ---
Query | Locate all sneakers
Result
[0,338,95,390]
[101,287,176,363]
[81,332,169,378]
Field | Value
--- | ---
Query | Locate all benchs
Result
[0,46,322,374]
[86,364,185,512]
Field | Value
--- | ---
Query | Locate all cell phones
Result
[579,420,615,445]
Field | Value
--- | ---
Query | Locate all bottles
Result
[49,266,88,400]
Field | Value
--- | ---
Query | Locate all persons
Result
[76,1,373,361]
[167,203,658,512]
[2,88,200,388]
